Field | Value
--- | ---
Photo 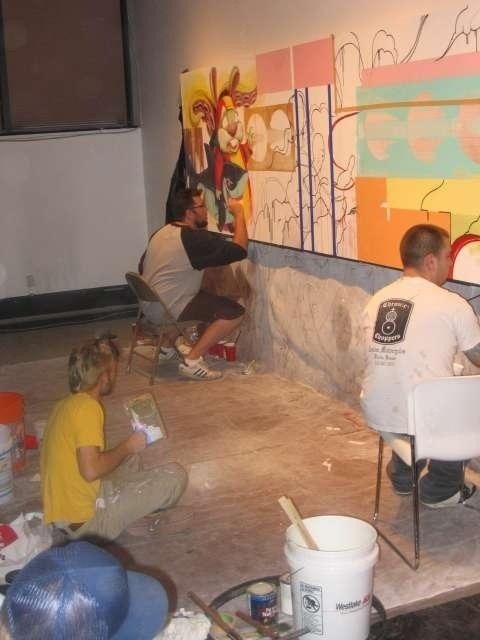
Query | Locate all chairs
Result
[371,365,479,570]
[120,269,206,390]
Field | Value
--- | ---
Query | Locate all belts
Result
[56,522,87,537]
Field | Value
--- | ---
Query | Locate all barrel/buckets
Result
[0,426,14,505]
[283,514,381,636]
[1,391,27,470]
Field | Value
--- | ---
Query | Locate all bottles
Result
[191,327,197,344]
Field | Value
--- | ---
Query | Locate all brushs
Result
[240,360,255,375]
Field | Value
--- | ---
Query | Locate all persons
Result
[37,338,188,551]
[354,222,480,509]
[136,186,249,383]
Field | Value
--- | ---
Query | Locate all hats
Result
[2,538,171,640]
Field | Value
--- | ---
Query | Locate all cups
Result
[223,343,236,362]
[33,420,48,448]
[216,341,227,359]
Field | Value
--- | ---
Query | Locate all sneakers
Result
[417,481,477,511]
[393,482,415,497]
[151,346,185,366]
[177,360,223,381]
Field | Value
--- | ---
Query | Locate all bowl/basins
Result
[207,612,235,638]
